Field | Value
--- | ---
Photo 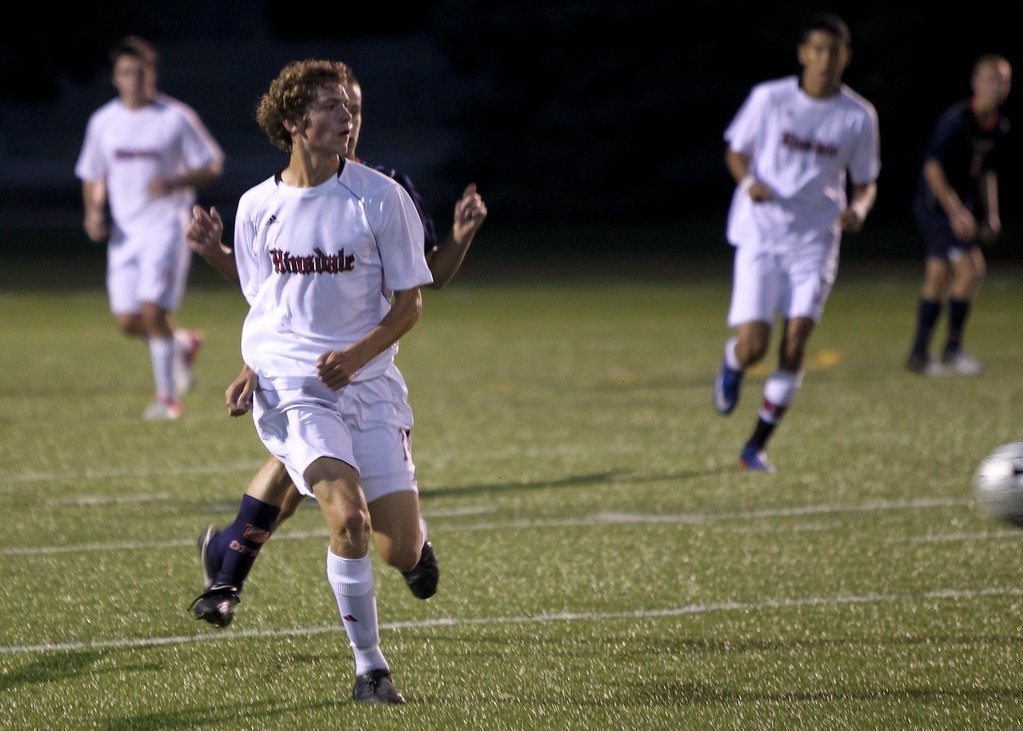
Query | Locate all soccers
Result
[970,440,1023,521]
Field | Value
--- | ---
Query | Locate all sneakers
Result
[353,669,407,705]
[187,579,241,626]
[402,539,438,601]
[199,526,220,593]
[714,365,742,415]
[739,443,774,474]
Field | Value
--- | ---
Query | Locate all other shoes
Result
[909,354,943,377]
[144,399,182,421]
[178,332,202,401]
[942,349,981,376]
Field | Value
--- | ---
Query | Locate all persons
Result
[186,71,487,626]
[914,55,1012,379]
[75,40,224,422]
[712,16,881,474]
[231,60,440,708]
